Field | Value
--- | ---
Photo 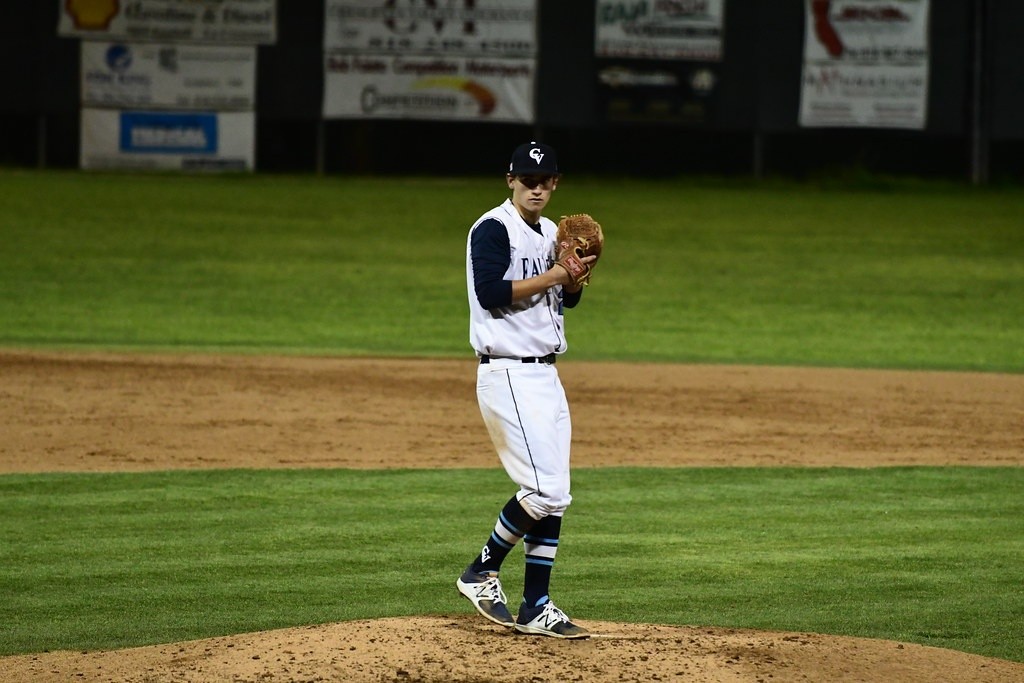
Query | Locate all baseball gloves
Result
[555,212,605,272]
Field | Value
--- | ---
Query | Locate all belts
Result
[480,352,557,366]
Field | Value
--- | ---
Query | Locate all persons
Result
[456,142,599,640]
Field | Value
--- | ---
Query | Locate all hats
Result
[508,140,563,176]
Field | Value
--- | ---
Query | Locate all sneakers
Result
[455,563,516,628]
[515,599,590,640]
